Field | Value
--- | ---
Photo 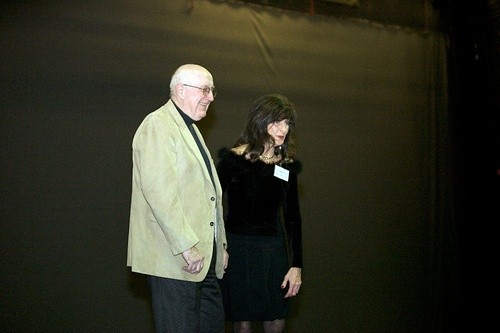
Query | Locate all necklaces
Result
[260,151,275,160]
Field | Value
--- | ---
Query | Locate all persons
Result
[127,64,229,333]
[216,94,303,333]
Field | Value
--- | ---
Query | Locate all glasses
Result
[183,84,217,98]
